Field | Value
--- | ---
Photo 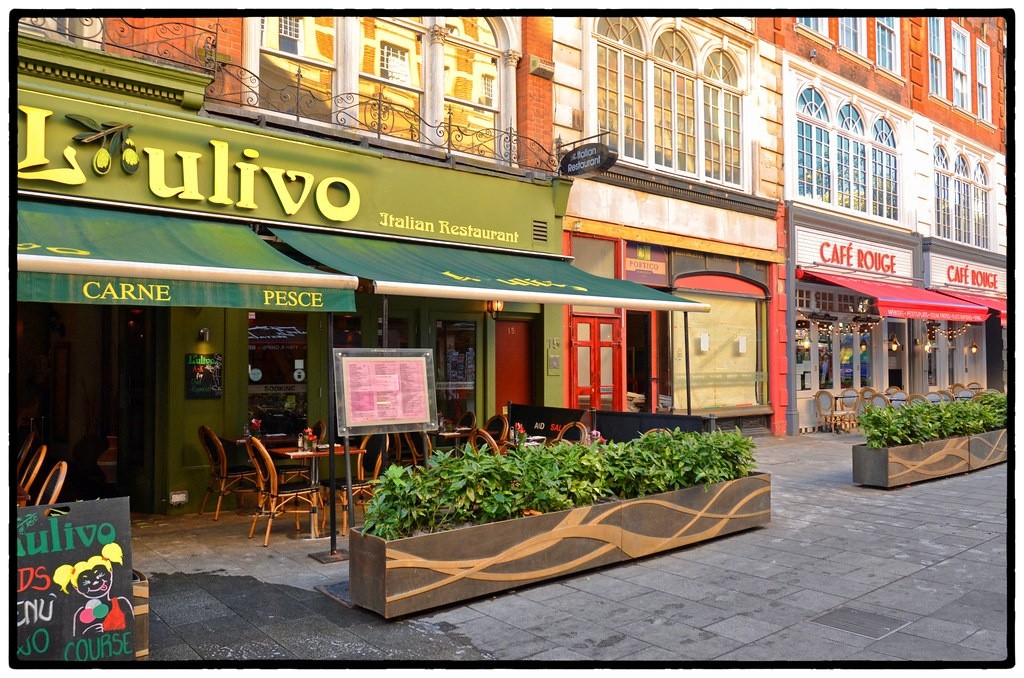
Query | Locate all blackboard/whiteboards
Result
[17,496,136,661]
[185,353,223,400]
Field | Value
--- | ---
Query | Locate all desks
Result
[834,396,857,431]
[889,399,906,403]
[429,431,499,458]
[955,396,972,399]
[220,436,367,538]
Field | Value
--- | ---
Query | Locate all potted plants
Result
[850,392,1009,488]
[349,425,771,619]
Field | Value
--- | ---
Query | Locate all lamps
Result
[970,342,979,354]
[487,301,503,320]
[891,333,901,351]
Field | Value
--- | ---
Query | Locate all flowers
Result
[514,422,527,443]
[304,427,317,451]
[590,428,606,442]
[250,419,261,432]
[444,418,453,431]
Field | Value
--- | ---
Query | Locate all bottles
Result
[510,426,514,439]
[298,433,304,447]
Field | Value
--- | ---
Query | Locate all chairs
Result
[317,427,390,536]
[385,409,587,469]
[267,423,327,515]
[14,431,67,516]
[817,382,1001,436]
[198,426,266,521]
[246,436,320,547]
